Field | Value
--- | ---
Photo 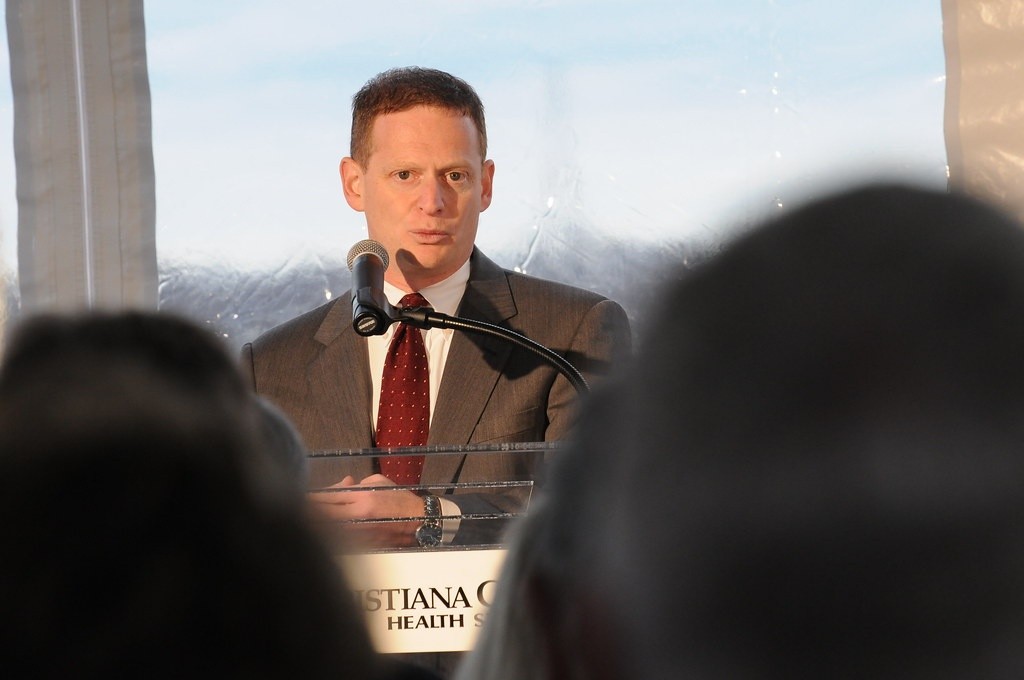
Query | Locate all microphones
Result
[347,239,390,336]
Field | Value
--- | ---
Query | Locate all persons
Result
[455,182,1024,680]
[238,68,632,548]
[0,312,433,680]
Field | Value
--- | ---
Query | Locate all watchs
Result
[415,493,442,548]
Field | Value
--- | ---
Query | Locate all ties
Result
[373,293,429,496]
